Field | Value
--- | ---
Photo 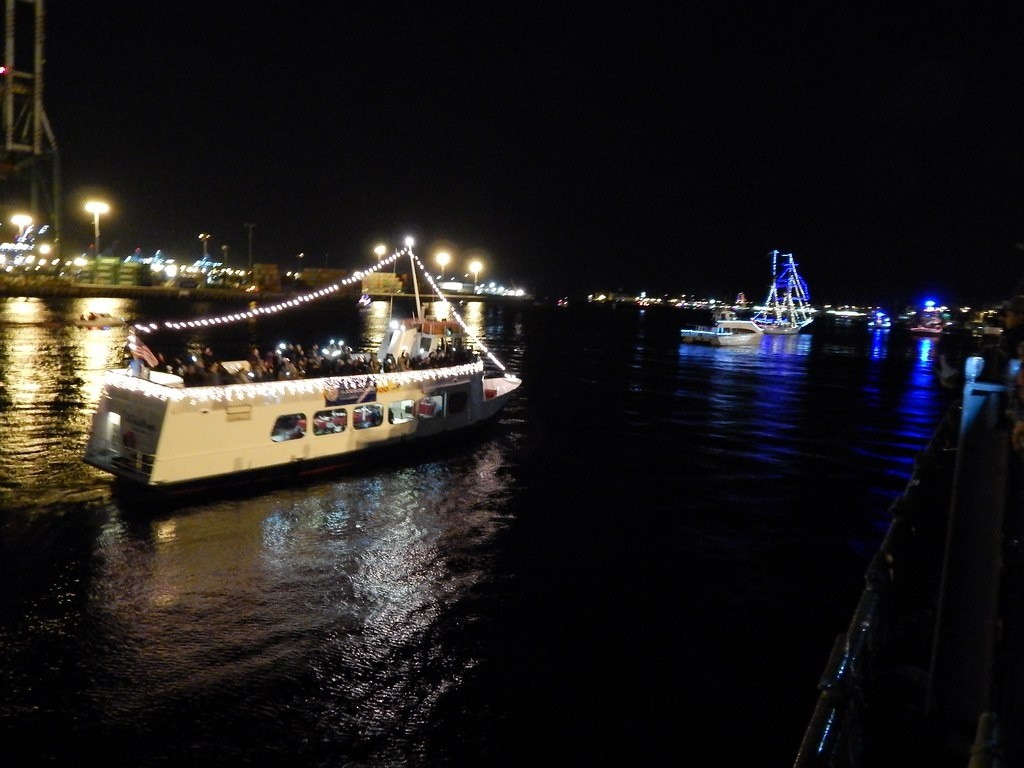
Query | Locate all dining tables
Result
[272,400,436,442]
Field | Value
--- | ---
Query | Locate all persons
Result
[273,404,394,439]
[134,340,480,388]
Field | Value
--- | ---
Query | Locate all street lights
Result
[9,199,485,290]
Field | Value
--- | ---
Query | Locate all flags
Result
[130,335,158,367]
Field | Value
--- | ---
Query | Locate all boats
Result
[749,249,817,334]
[83,236,521,488]
[862,297,950,332]
[678,292,764,343]
